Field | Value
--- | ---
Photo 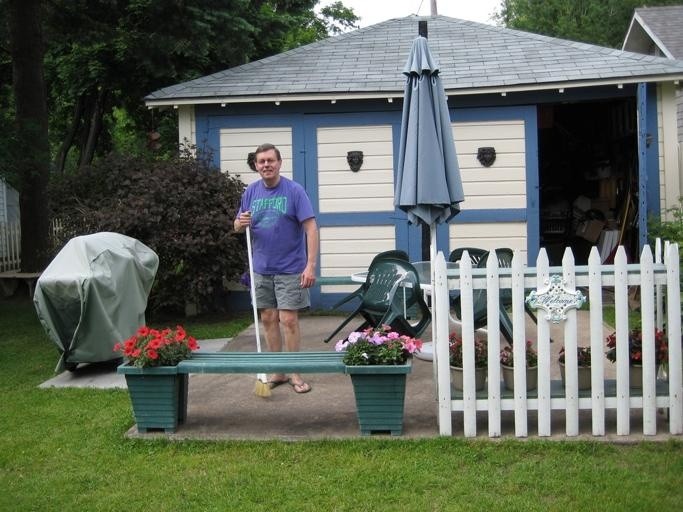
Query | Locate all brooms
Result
[245,225,272,394]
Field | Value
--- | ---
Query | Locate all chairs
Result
[453,247,553,352]
[330,249,409,311]
[324,258,432,343]
[448,247,488,266]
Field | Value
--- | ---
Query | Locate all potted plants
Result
[558,346,591,391]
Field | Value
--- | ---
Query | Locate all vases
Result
[116,361,188,434]
[344,359,413,437]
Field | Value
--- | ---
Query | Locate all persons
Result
[234,143,319,394]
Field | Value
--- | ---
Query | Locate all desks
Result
[351,261,477,361]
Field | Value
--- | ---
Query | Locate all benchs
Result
[180,350,346,373]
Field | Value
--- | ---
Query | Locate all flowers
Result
[449,330,488,368]
[607,325,669,366]
[336,325,425,365]
[113,324,198,364]
[502,339,537,368]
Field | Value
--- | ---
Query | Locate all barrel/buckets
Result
[557,359,592,389]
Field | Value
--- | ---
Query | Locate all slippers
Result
[267,378,289,389]
[289,377,311,393]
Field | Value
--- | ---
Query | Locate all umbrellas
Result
[392,36,466,424]
